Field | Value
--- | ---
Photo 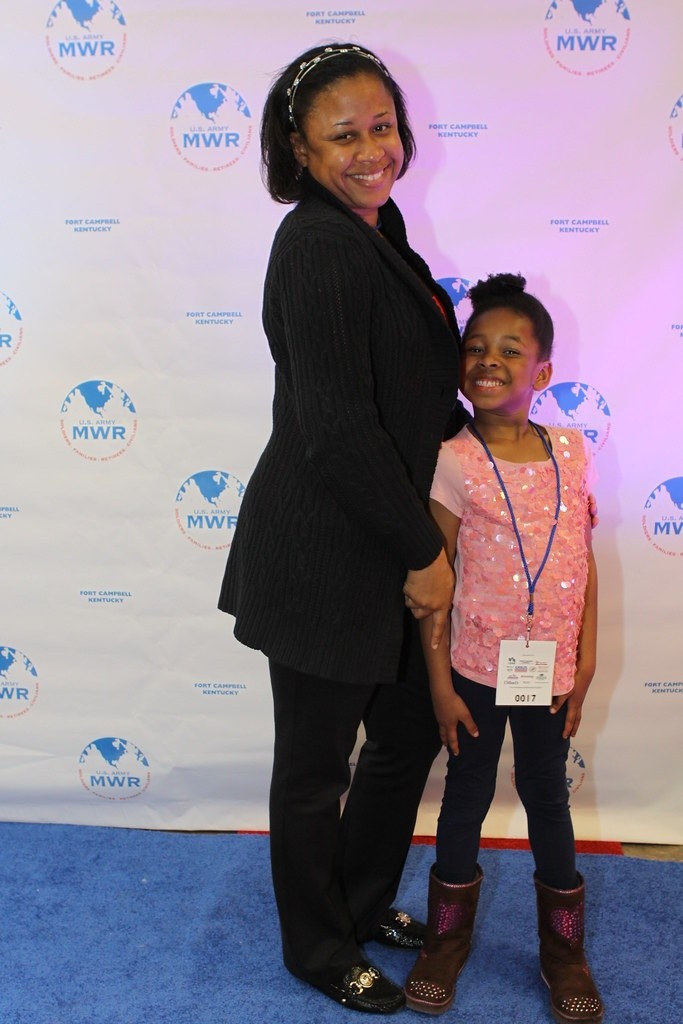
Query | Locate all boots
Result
[531,868,604,1024]
[404,861,484,1014]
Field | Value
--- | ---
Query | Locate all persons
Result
[217,43,476,1015]
[403,272,604,1024]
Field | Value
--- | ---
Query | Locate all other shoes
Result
[317,960,405,1014]
[369,907,429,950]
